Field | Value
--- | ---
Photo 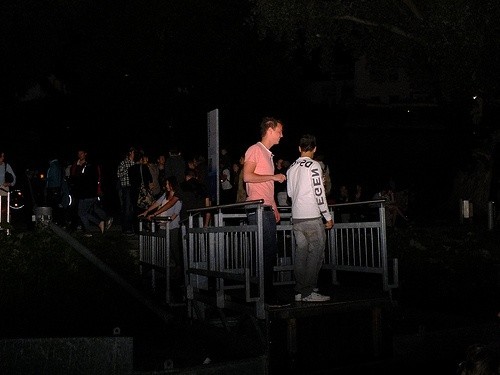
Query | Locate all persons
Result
[0,149,407,267]
[242,117,291,308]
[286,136,334,302]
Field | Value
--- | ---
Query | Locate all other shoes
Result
[83,233,92,237]
[266,298,291,308]
[100,221,104,234]
[105,217,113,232]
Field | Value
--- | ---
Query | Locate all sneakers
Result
[295,294,302,302]
[302,291,331,302]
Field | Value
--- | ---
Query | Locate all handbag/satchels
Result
[4,163,13,182]
[137,185,156,210]
